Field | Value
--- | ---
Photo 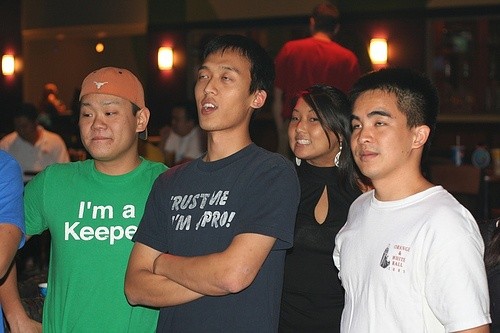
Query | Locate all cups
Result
[39,283,47,297]
[450,146,466,166]
[491,148,500,167]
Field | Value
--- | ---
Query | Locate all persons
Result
[0,149,26,333]
[124,34,301,333]
[332,67,493,333]
[0,67,172,333]
[277,85,375,333]
[272,4,362,161]
[0,83,94,275]
[135,97,206,170]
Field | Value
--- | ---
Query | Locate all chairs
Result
[420,136,500,218]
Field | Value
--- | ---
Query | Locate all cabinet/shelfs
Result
[420,1,499,123]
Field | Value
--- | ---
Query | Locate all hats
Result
[79,67,148,141]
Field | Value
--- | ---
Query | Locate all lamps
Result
[1,55,14,76]
[158,46,174,71]
[368,38,387,65]
